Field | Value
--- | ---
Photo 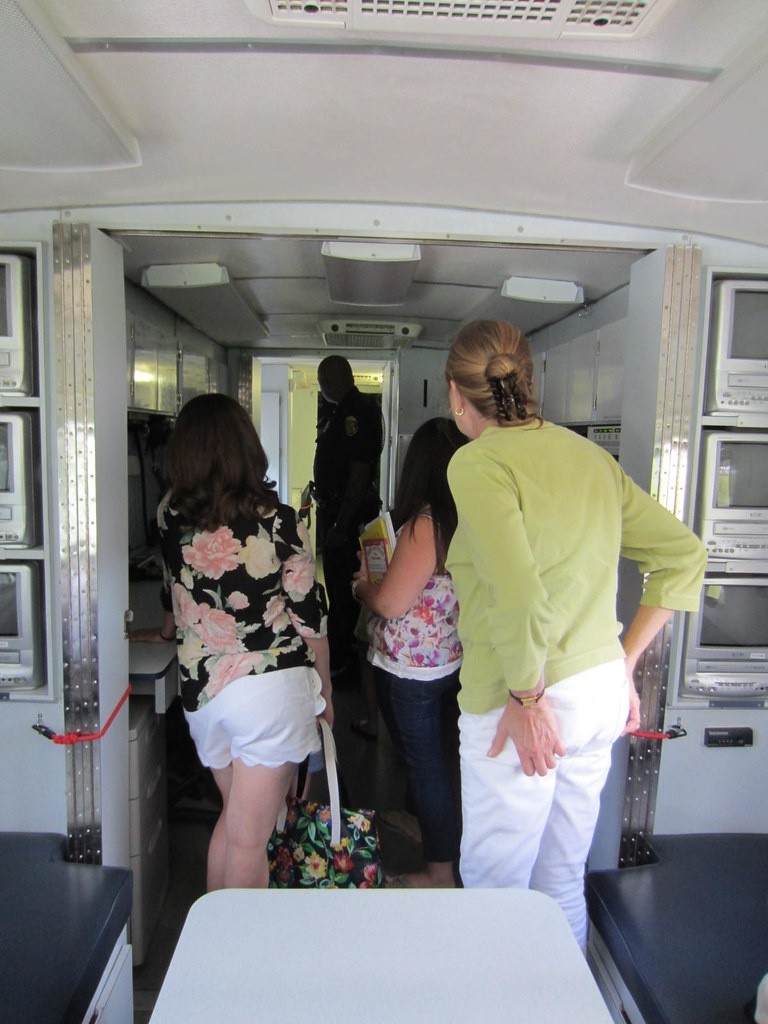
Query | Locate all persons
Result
[156,393,333,892]
[314,354,384,672]
[355,417,468,888]
[446,319,708,957]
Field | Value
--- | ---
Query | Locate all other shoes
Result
[331,671,360,690]
[351,719,390,741]
[385,874,408,888]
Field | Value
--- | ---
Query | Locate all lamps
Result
[445,275,584,342]
[140,263,271,343]
[320,241,421,310]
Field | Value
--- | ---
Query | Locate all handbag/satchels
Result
[266,716,382,888]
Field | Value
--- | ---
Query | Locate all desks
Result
[148,888,616,1024]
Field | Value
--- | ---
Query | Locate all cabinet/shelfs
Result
[82,923,133,1024]
[587,921,646,1024]
[127,281,230,418]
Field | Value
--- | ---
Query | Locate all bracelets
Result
[351,577,367,601]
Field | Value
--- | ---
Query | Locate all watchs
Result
[509,689,545,706]
